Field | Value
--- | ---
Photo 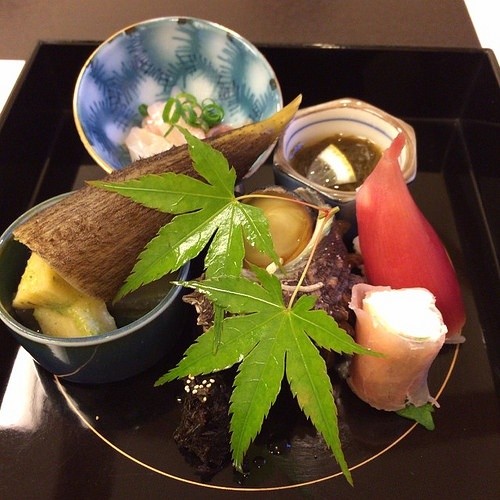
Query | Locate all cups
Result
[275,96,418,230]
[72,14,284,190]
[0,190,196,385]
